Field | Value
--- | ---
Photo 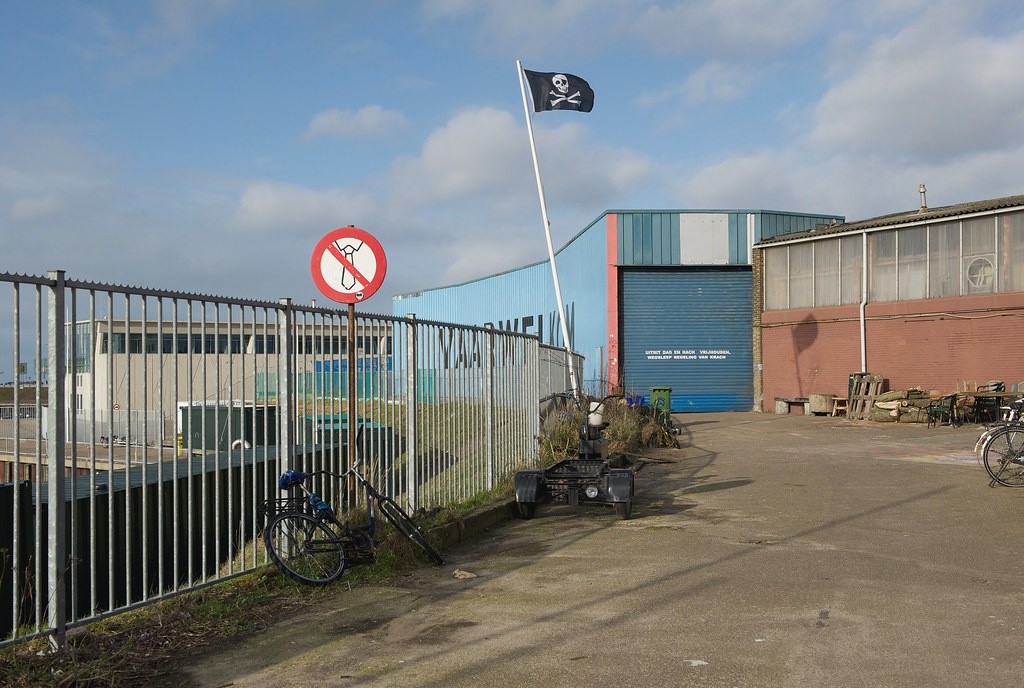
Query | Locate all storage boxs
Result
[0,402,393,632]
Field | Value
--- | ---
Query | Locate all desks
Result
[974,392,1024,427]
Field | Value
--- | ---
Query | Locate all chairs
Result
[919,382,1005,428]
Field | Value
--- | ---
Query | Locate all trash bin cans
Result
[649,385,672,420]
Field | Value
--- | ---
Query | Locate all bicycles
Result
[973,398,1024,481]
[256,424,445,586]
[981,402,1024,489]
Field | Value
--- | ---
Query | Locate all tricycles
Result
[514,393,634,520]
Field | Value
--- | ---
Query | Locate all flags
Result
[524,69,594,112]
